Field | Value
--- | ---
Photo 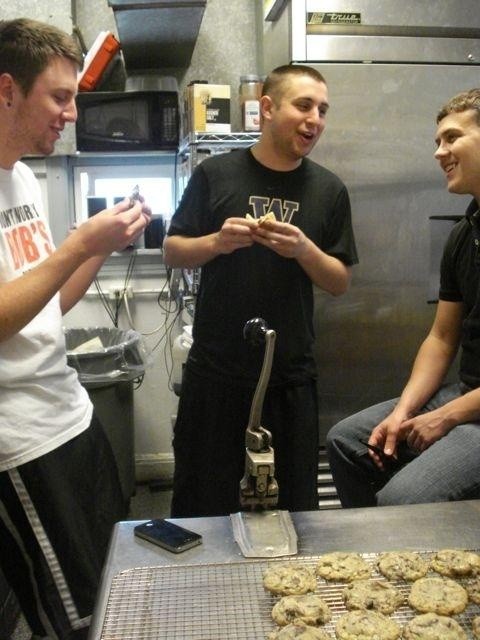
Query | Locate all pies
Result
[263,547,480,640]
[245,211,276,228]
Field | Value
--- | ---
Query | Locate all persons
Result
[160,63,360,515]
[0,16,151,640]
[323,89,480,506]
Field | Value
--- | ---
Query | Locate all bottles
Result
[239,73,262,131]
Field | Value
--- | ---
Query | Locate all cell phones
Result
[133,517,204,553]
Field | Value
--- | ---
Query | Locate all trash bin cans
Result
[63,326,141,511]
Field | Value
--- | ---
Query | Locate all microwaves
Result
[75,89,181,152]
